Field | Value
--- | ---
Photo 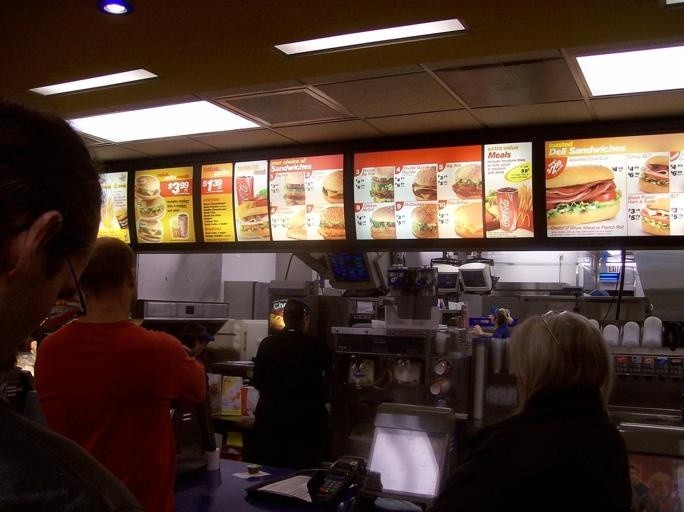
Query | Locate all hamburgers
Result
[637,154,670,235]
[546,165,623,223]
[369,164,482,238]
[284,170,345,240]
[238,189,270,240]
[136,175,165,243]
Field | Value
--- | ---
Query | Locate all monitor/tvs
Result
[365,402,456,506]
[458,263,493,291]
[325,253,382,296]
[431,262,459,293]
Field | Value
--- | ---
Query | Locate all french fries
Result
[516,183,533,232]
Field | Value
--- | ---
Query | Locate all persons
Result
[505,309,517,327]
[177,323,214,451]
[245,298,341,465]
[0,113,145,512]
[429,310,632,512]
[35,236,207,512]
[473,308,508,339]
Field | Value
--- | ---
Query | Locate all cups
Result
[430,361,451,396]
[247,465,262,475]
[205,448,222,471]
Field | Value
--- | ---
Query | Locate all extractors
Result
[140,301,230,336]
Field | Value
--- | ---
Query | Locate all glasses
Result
[540,309,567,346]
[40,237,87,335]
